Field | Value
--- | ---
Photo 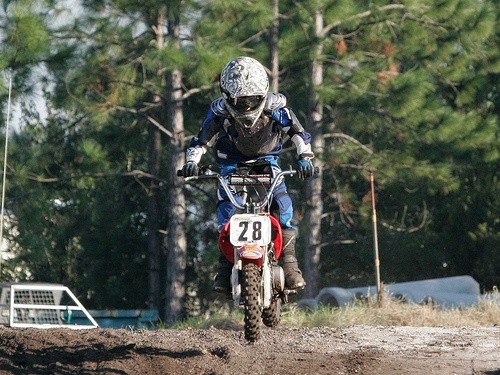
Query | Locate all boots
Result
[282,229,307,288]
[212,251,233,298]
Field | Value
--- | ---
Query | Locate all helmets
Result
[220,57,269,128]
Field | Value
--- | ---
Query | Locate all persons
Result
[181,57,315,291]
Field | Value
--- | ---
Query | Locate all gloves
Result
[297,159,314,180]
[182,162,198,181]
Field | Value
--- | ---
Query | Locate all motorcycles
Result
[176,165,322,345]
